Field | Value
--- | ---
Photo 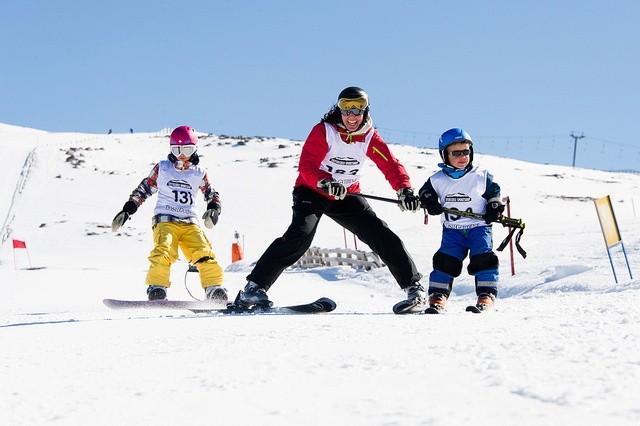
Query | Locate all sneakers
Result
[147,286,168,300]
[476,293,496,311]
[407,283,426,306]
[429,293,447,314]
[240,280,268,301]
[206,287,228,300]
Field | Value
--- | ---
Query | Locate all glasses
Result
[341,108,365,116]
[448,149,471,156]
[170,145,196,157]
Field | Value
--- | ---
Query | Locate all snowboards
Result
[104,299,228,310]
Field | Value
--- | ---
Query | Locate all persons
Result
[110,126,229,300]
[235,87,424,313]
[418,129,506,313]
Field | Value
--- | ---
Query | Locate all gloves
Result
[317,178,347,200]
[482,201,505,224]
[396,187,420,212]
[421,191,443,215]
[202,202,221,229]
[112,201,138,232]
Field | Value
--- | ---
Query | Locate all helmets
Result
[438,128,473,170]
[338,87,368,110]
[170,126,198,145]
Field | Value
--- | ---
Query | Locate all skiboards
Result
[224,298,418,314]
[426,304,481,314]
